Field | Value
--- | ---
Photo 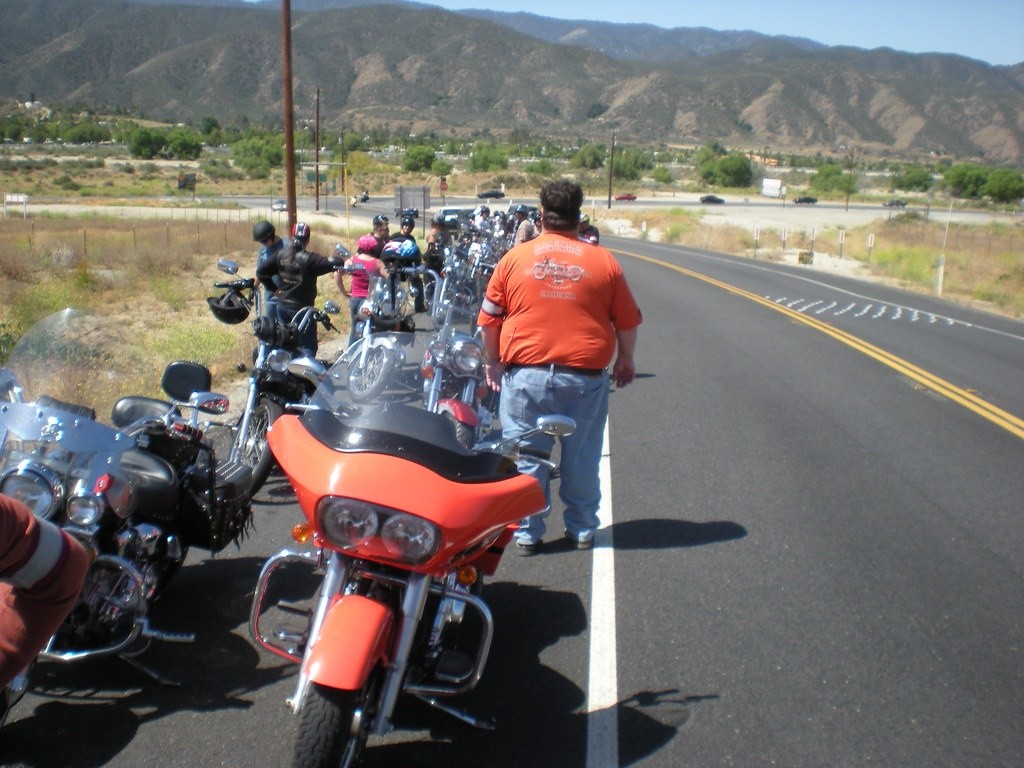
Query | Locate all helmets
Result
[580,214,590,223]
[253,221,276,241]
[401,217,416,231]
[432,204,542,228]
[372,214,389,229]
[357,235,377,252]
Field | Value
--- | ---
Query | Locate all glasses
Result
[207,296,252,323]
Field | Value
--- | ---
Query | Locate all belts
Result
[515,363,603,376]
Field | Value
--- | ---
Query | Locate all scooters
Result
[349,194,369,208]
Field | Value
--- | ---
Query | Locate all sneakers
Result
[517,539,542,555]
[564,528,592,549]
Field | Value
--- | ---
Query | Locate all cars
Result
[700,195,724,204]
[271,199,287,212]
[882,198,908,208]
[394,206,419,219]
[440,207,462,229]
[615,193,637,202]
[477,189,505,201]
[794,195,817,205]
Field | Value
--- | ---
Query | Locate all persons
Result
[476,180,642,556]
[427,204,600,300]
[350,190,369,207]
[334,215,427,347]
[251,220,344,359]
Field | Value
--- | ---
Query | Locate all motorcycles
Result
[0,307,255,739]
[249,329,577,768]
[203,260,341,502]
[332,200,599,452]
